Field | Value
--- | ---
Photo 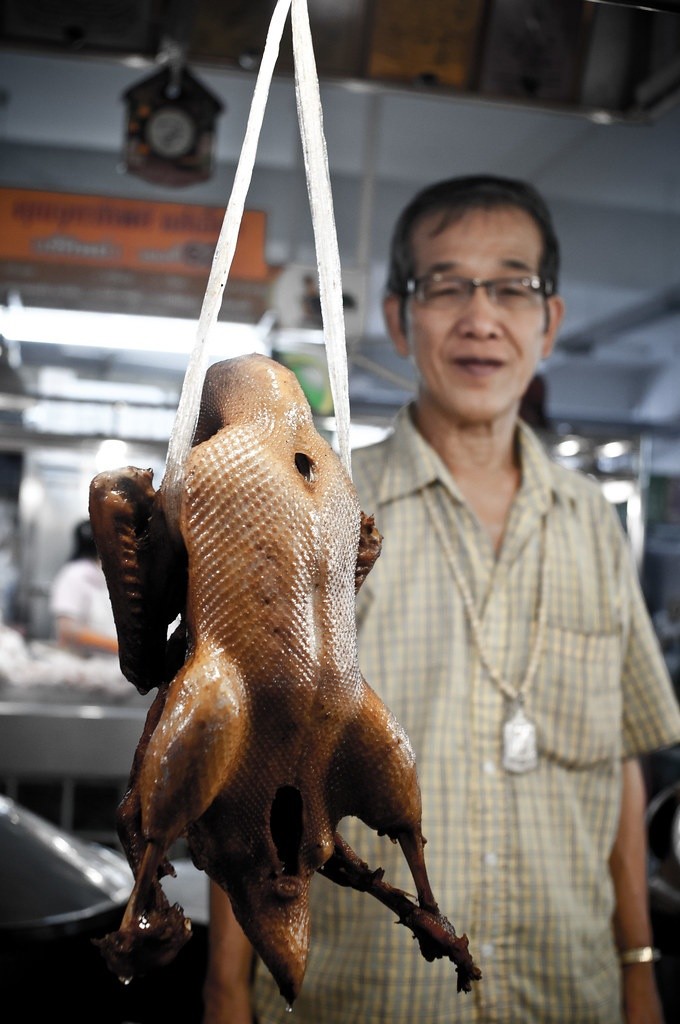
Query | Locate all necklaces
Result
[422,487,551,776]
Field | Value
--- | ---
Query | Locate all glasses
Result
[405,275,551,310]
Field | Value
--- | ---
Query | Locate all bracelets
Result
[621,946,662,965]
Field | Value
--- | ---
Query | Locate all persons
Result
[49,520,118,662]
[205,173,680,1024]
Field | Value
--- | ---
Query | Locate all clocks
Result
[123,61,226,189]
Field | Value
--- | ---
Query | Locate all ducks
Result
[89,351,481,1001]
